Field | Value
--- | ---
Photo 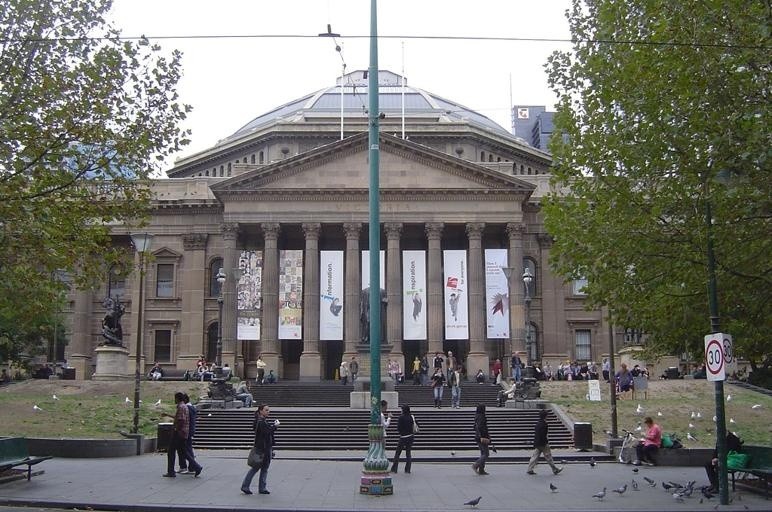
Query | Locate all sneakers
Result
[641,459,654,465]
[456,405,460,409]
[631,459,641,466]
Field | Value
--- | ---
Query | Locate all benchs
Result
[728,445,772,500]
[147,369,188,380]
[1,436,52,482]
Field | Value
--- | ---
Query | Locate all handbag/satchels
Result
[671,433,683,449]
[480,437,490,444]
[411,414,420,434]
[726,449,752,469]
[432,380,437,387]
[248,448,265,470]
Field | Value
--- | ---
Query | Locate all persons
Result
[240,404,279,494]
[448,290,462,321]
[631,417,661,466]
[381,400,419,474]
[385,351,522,409]
[195,354,276,385]
[526,409,564,475]
[160,393,202,478]
[703,427,744,495]
[150,362,163,381]
[543,357,706,392]
[472,403,491,476]
[411,293,423,321]
[329,298,342,317]
[340,356,358,385]
[236,380,256,407]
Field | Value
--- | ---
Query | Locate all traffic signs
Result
[719,334,735,374]
[702,333,726,385]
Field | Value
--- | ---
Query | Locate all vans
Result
[31,362,66,379]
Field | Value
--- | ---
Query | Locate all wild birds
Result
[549,482,558,492]
[657,408,662,416]
[463,495,482,507]
[450,451,456,456]
[126,396,132,403]
[602,429,611,435]
[492,444,497,453]
[589,457,596,467]
[636,403,644,413]
[686,393,760,442]
[155,398,162,406]
[53,394,59,400]
[591,467,656,501]
[661,479,750,511]
[139,399,143,404]
[34,404,43,411]
[634,421,641,431]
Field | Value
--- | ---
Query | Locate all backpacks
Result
[661,432,673,449]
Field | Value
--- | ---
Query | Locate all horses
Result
[101,297,121,345]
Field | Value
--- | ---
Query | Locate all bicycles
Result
[618,428,643,466]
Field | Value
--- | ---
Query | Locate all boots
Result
[434,399,437,408]
[472,463,479,473]
[438,400,441,409]
[478,467,489,475]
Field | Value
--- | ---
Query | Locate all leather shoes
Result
[706,485,714,491]
[554,467,563,475]
[710,488,718,493]
[241,488,252,494]
[526,470,536,474]
[259,490,270,494]
[163,465,203,477]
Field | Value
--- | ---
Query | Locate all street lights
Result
[131,230,155,435]
[213,267,228,386]
[502,265,516,378]
[602,239,625,454]
[520,266,537,379]
